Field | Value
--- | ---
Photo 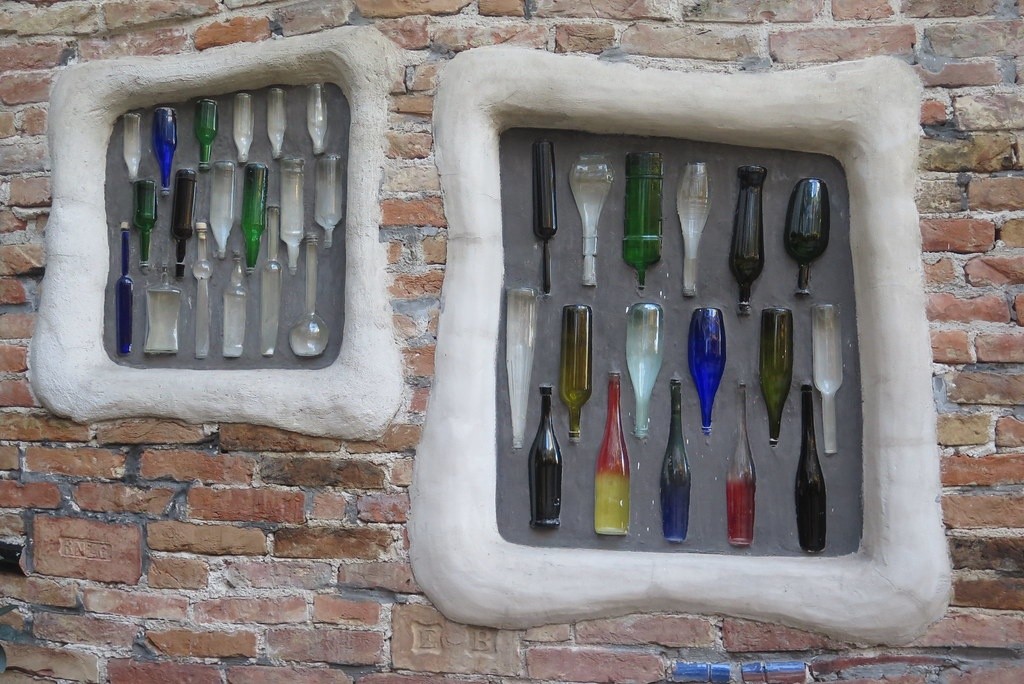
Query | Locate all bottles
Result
[795,384,826,551]
[115,79,343,355]
[594,372,630,535]
[783,178,830,295]
[659,377,691,542]
[675,161,712,297]
[560,304,592,442]
[758,308,792,445]
[811,303,842,453]
[531,139,558,295]
[728,166,767,316]
[687,308,726,434]
[625,303,664,438]
[621,151,663,290]
[568,154,614,286]
[505,288,537,449]
[529,385,563,527]
[726,382,757,546]
